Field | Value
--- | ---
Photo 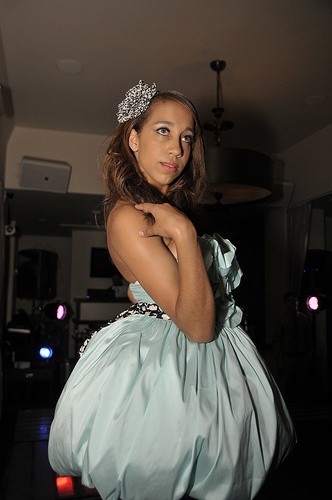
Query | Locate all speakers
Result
[19,155,71,194]
[17,250,58,299]
[298,249,332,312]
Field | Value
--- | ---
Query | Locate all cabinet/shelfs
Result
[73,297,134,361]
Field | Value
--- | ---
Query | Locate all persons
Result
[48,79,297,500]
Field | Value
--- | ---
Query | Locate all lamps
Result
[7,308,32,333]
[306,293,329,314]
[198,60,273,205]
